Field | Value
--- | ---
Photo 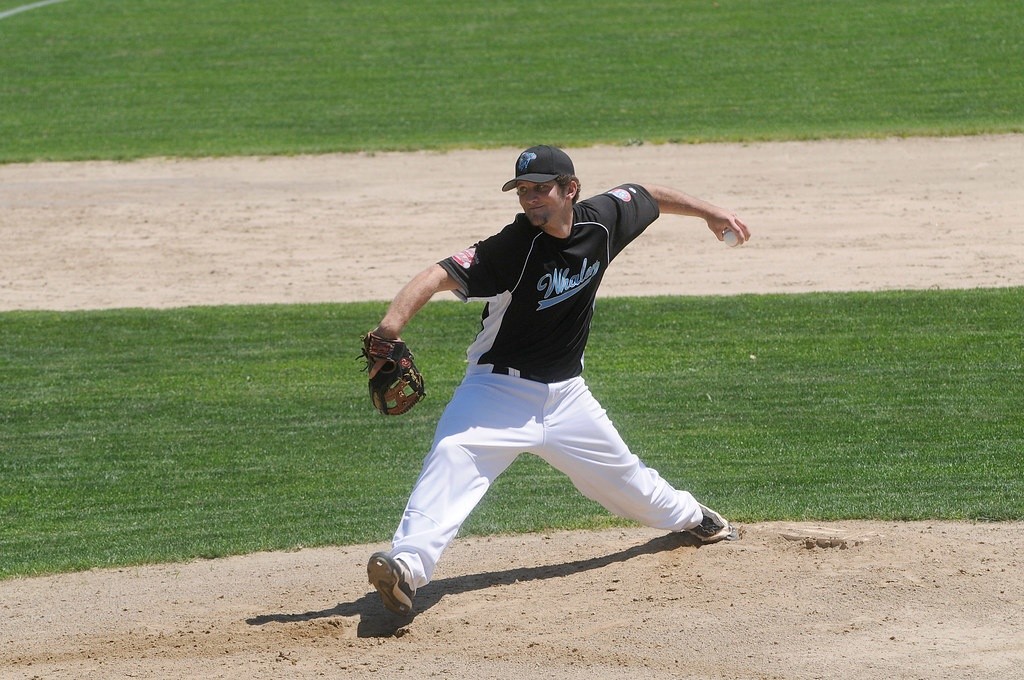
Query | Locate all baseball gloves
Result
[360,332,426,417]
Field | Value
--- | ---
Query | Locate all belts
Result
[492,365,566,383]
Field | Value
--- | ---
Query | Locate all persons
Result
[360,145,752,614]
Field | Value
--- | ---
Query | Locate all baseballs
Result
[723,228,741,248]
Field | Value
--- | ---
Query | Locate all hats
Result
[501,144,575,192]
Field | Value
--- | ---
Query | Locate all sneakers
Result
[367,552,414,617]
[688,510,728,541]
